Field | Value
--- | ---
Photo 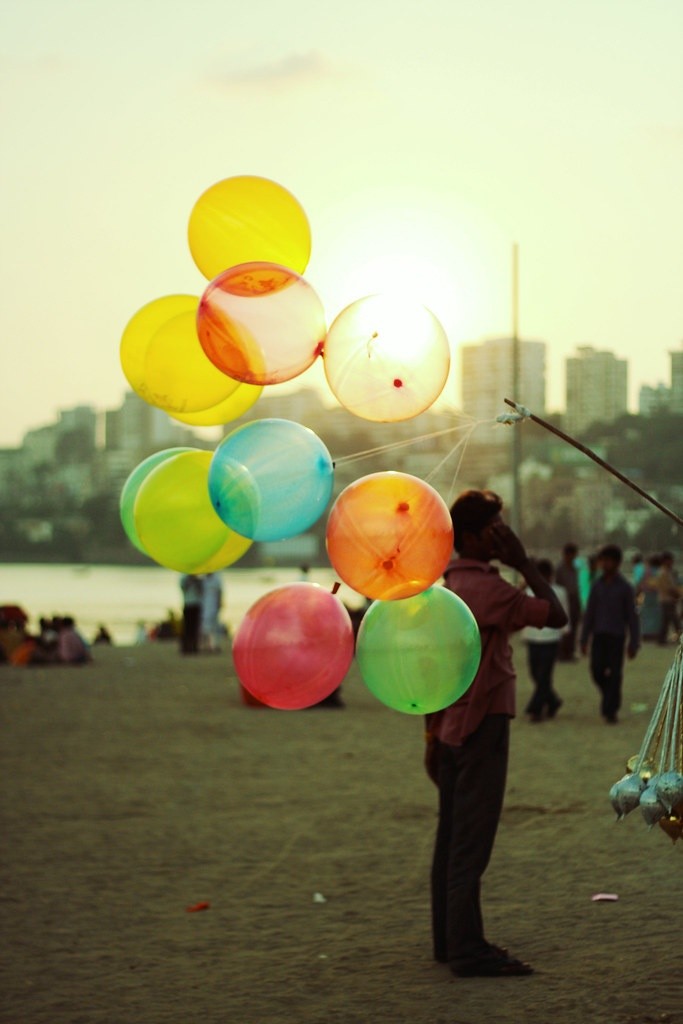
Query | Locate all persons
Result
[96,628,111,643]
[0,605,92,664]
[632,552,683,644]
[181,573,222,652]
[519,543,640,723]
[424,491,570,977]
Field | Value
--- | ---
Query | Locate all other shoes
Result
[524,711,542,720]
[438,947,537,975]
[546,700,562,718]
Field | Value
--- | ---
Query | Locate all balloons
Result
[197,262,326,386]
[326,470,455,601]
[355,581,481,714]
[209,416,336,542]
[187,174,312,298]
[323,294,450,422]
[122,293,267,427]
[233,585,354,709]
[119,447,259,572]
